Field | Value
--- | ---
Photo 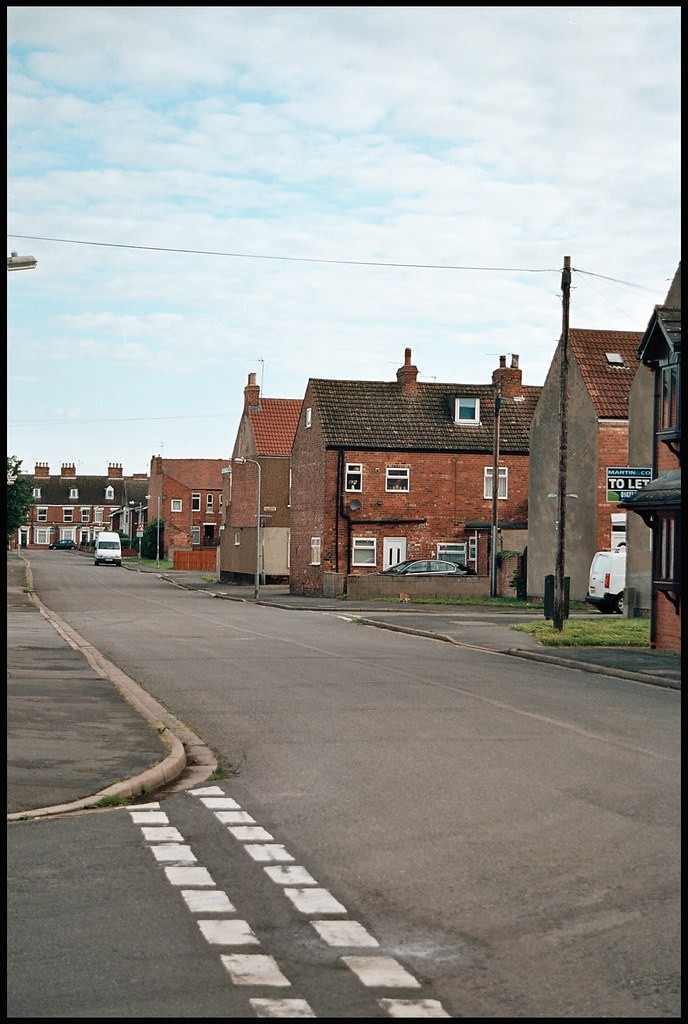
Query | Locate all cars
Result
[48,539,77,550]
[584,542,627,614]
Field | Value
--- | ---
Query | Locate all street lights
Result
[128,501,142,558]
[233,457,261,597]
[144,495,160,568]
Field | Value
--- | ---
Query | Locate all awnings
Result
[616,470,681,527]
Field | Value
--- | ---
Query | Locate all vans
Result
[94,531,122,566]
